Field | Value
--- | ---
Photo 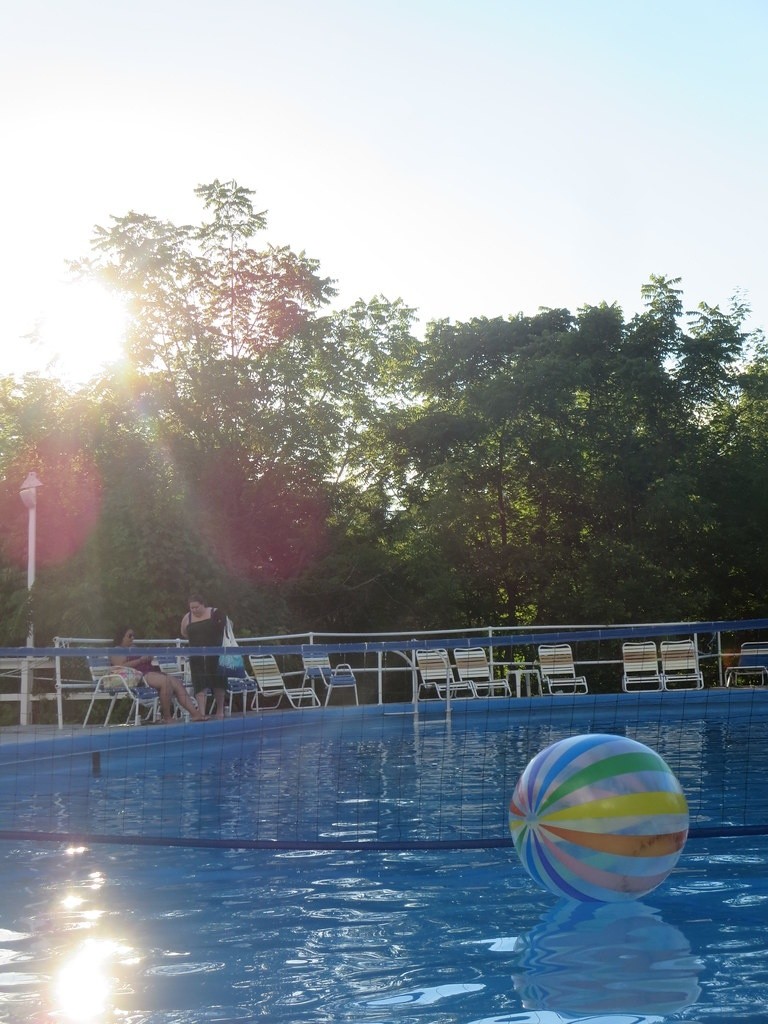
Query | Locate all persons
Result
[110,625,212,724]
[181,594,229,720]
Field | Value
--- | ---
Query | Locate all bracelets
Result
[140,657,145,664]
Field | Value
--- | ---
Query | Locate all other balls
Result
[506,734,689,906]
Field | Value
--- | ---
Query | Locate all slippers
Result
[191,715,209,722]
[142,719,166,725]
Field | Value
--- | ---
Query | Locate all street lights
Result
[18,469,46,726]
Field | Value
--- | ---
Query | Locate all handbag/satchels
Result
[217,616,246,677]
[102,665,142,688]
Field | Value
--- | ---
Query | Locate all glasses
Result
[128,634,135,638]
